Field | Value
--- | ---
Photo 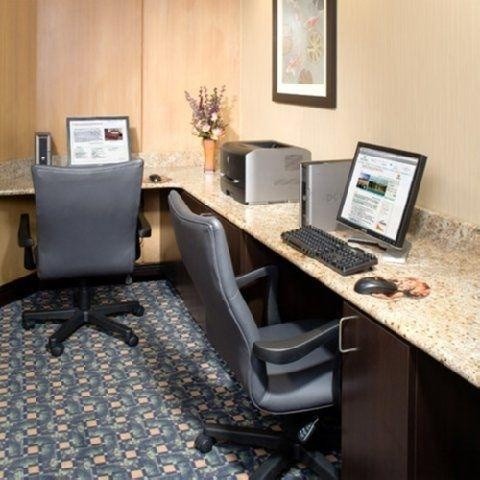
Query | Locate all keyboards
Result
[281,225,378,276]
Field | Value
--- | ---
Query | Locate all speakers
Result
[36,132,50,166]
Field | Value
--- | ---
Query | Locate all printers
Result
[220,140,311,204]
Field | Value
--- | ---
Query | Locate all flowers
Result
[183,82,231,144]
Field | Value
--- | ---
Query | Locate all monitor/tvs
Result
[66,116,132,166]
[336,141,427,264]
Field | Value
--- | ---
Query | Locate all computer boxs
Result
[300,159,353,230]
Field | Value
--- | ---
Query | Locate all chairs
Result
[15,154,150,359]
[165,185,348,480]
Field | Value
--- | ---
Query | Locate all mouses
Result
[150,175,161,182]
[354,277,396,294]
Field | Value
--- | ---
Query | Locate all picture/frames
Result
[267,0,339,113]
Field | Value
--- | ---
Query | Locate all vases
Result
[202,138,218,175]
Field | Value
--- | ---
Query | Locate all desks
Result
[159,149,479,480]
[0,163,179,201]
